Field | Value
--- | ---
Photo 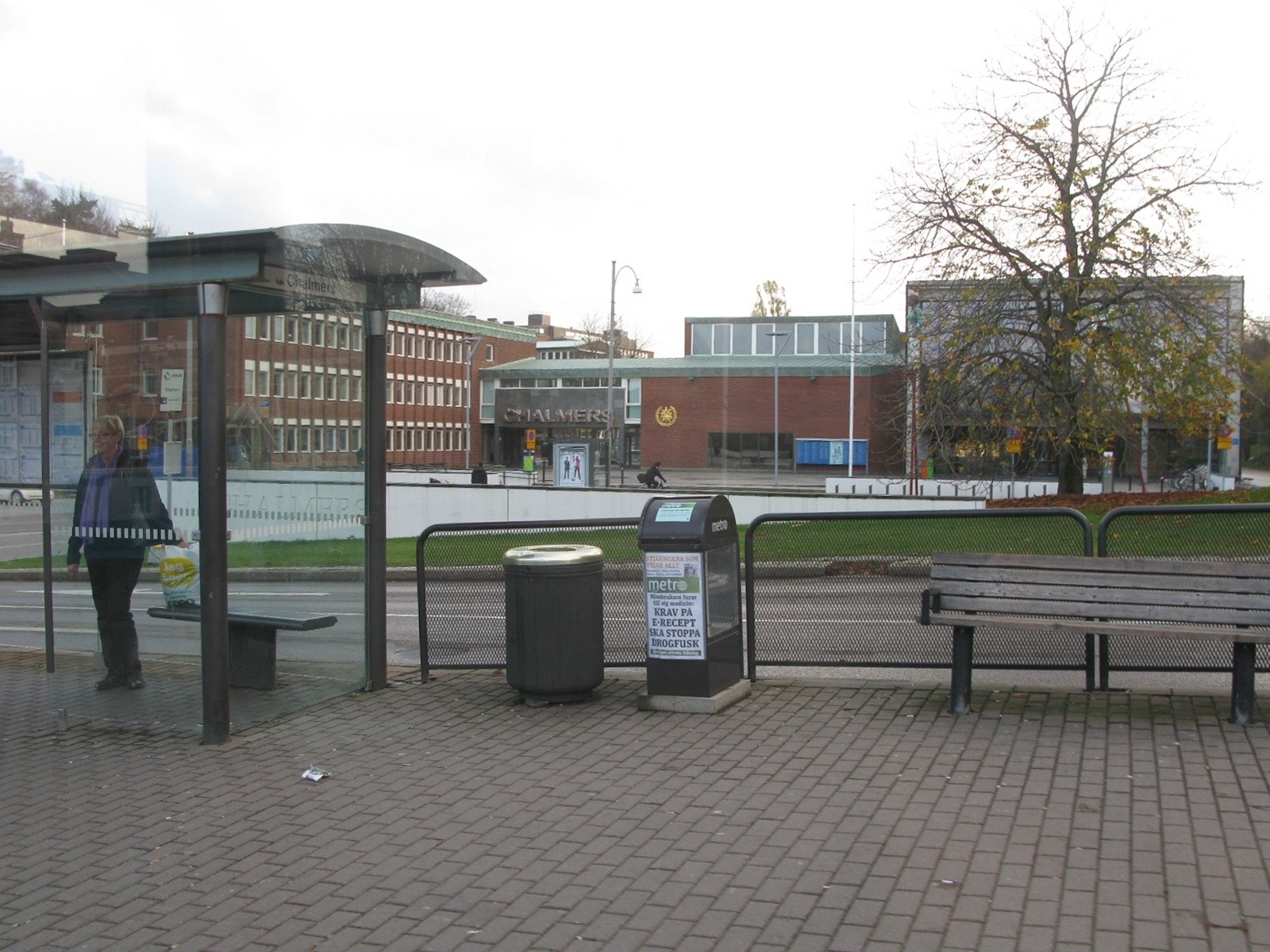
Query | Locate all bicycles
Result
[638,480,671,489]
[1174,464,1215,492]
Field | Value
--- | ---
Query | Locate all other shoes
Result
[94,667,128,690]
[127,670,145,689]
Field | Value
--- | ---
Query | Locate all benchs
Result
[915,551,1270,724]
[147,604,340,690]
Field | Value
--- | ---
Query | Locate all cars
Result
[0,489,55,506]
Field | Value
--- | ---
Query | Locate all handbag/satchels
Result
[154,541,201,608]
[637,473,646,483]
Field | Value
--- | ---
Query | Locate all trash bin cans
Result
[636,492,744,697]
[502,544,605,706]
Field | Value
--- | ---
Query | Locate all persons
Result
[645,461,666,489]
[63,413,184,691]
[470,461,489,485]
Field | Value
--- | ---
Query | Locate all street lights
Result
[765,331,793,485]
[605,260,642,487]
[459,338,482,471]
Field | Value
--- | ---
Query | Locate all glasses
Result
[89,430,116,440]
